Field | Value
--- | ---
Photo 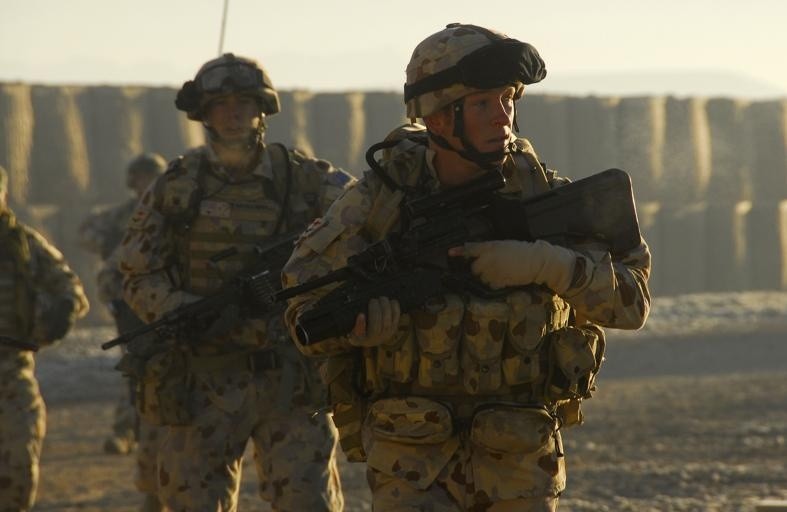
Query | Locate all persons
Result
[83,152,169,452]
[283,21,652,512]
[0,168,91,511]
[112,51,356,511]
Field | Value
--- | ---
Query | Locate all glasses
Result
[192,60,264,94]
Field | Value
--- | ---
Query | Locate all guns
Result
[102,240,294,348]
[272,170,640,346]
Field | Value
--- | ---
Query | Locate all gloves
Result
[348,296,403,352]
[466,238,577,298]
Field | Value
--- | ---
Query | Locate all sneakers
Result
[102,428,139,455]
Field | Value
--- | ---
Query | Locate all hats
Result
[404,22,546,121]
[173,55,282,123]
[125,151,167,188]
